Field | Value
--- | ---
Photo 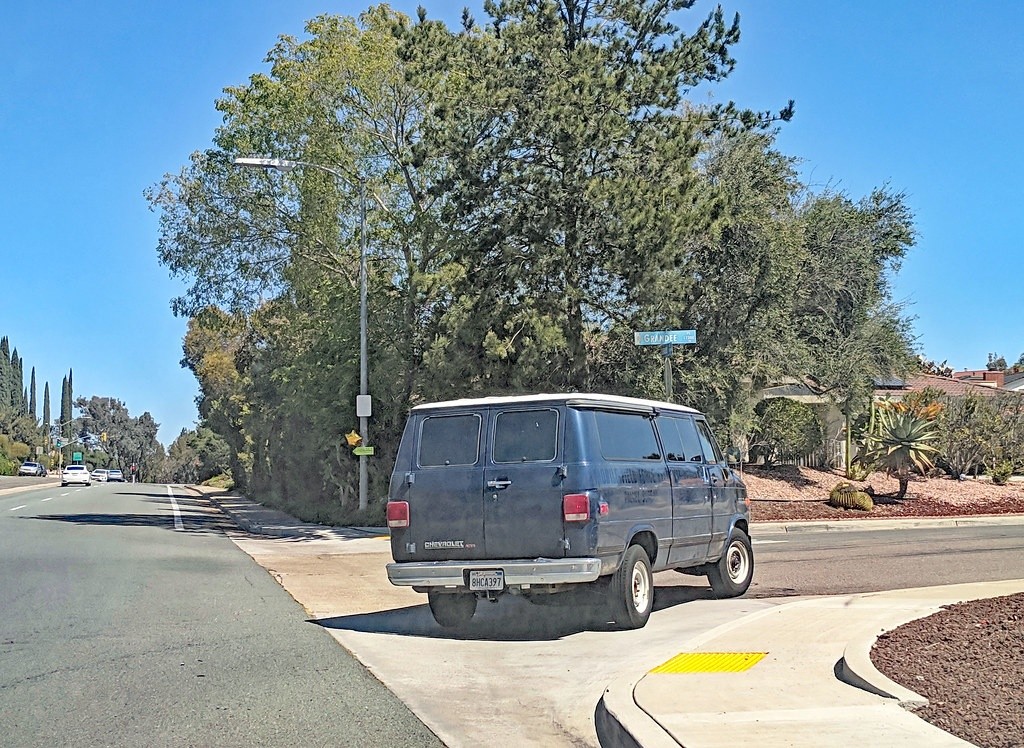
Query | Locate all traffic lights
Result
[131,466,136,475]
[57,439,61,447]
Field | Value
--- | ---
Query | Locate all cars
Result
[18,462,42,477]
[108,470,124,482]
[40,465,47,478]
[62,465,92,487]
[91,468,110,482]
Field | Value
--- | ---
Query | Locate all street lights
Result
[231,156,380,515]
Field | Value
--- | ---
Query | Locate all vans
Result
[384,393,755,632]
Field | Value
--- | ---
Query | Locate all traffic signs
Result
[351,445,375,456]
